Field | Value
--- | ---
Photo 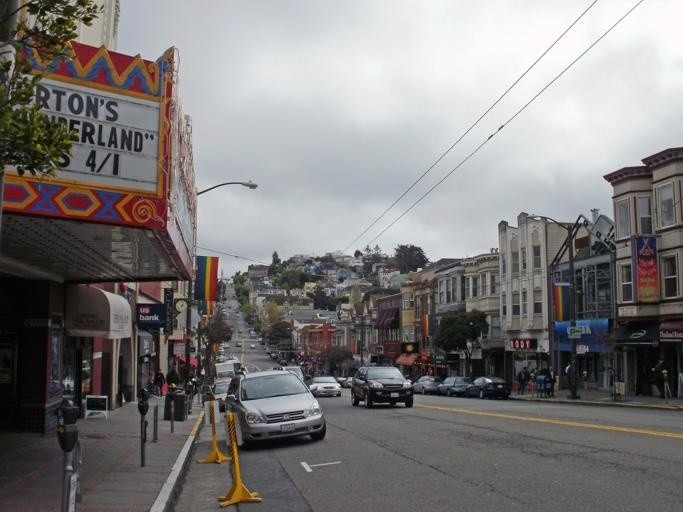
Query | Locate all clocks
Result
[175,299,188,313]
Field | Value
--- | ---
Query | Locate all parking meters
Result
[530,373,535,397]
[195,378,202,404]
[168,383,176,433]
[186,382,193,414]
[137,389,149,469]
[583,372,587,399]
[56,399,78,512]
[662,370,668,404]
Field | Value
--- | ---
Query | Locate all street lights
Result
[406,281,438,378]
[526,215,575,395]
[184,181,257,388]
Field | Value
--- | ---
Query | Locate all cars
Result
[437,377,472,396]
[224,370,326,450]
[350,367,413,408]
[309,376,352,397]
[213,341,243,411]
[466,377,510,399]
[264,345,287,365]
[412,375,441,394]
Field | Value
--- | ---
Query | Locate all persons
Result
[180,365,192,384]
[565,361,573,387]
[515,364,555,398]
[652,358,671,397]
[166,366,180,393]
[153,368,165,397]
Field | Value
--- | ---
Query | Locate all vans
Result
[272,366,303,382]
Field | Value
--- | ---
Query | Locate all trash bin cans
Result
[164,386,184,421]
[535,375,552,398]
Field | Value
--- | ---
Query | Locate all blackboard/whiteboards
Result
[85,395,107,412]
